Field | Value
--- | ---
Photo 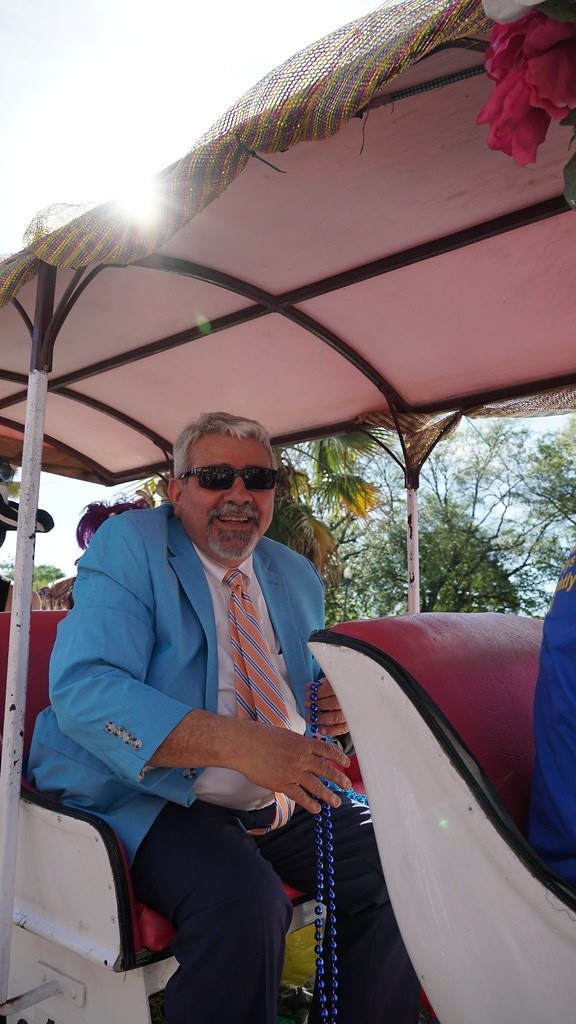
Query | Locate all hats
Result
[0,459,54,533]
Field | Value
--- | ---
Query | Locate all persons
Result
[0,458,151,613]
[528,547,575,887]
[27,412,422,1024]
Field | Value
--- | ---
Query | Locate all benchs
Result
[0,609,368,973]
[306,612,576,1024]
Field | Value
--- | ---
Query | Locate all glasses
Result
[179,464,279,491]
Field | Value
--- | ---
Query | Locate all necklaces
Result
[309,681,338,1024]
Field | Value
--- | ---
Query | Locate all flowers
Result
[473,0,576,211]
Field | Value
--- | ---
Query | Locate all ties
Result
[223,568,297,834]
